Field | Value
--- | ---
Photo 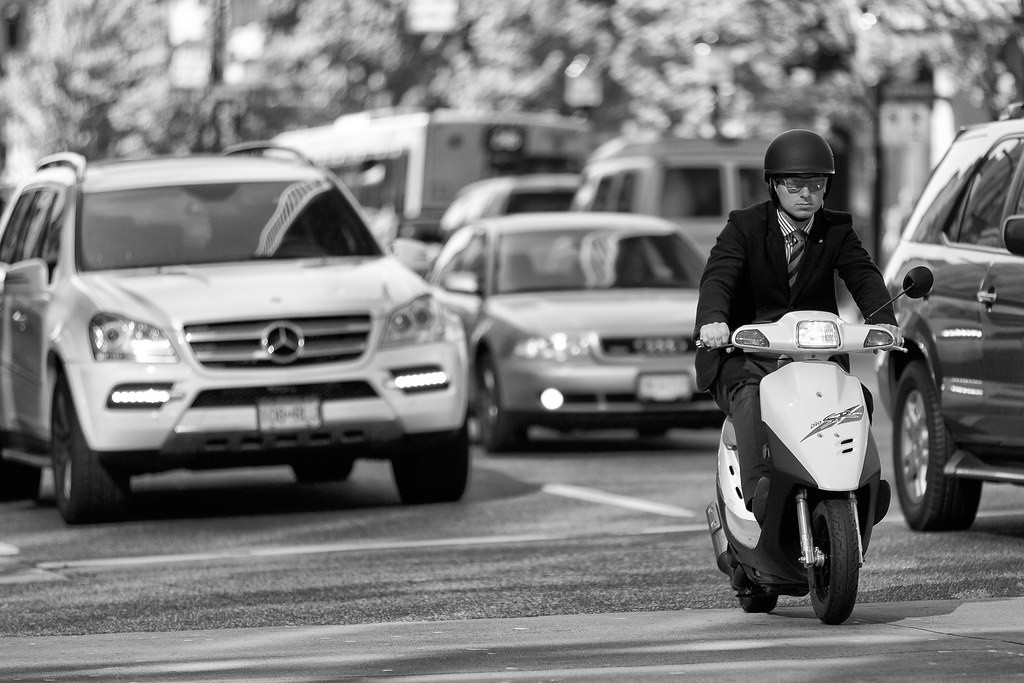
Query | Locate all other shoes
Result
[752,477,772,530]
[873,480,891,525]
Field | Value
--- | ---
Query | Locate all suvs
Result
[869,99,1024,533]
[0,141,467,530]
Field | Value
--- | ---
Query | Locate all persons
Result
[696,129,904,528]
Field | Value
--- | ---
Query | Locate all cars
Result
[399,171,578,273]
[425,214,708,456]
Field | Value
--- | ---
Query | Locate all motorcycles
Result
[695,267,934,625]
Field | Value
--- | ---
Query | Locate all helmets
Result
[764,130,835,174]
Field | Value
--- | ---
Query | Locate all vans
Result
[575,134,773,268]
[259,105,589,241]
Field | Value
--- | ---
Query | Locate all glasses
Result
[776,177,827,193]
